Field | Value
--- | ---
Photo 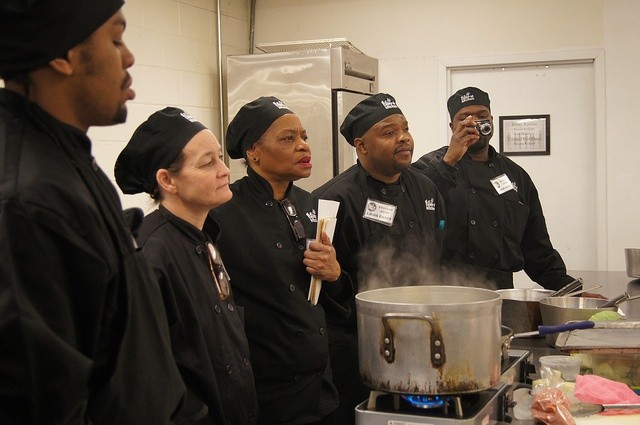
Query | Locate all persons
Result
[0,0,209,425]
[408,86,608,300]
[113,105,261,425]
[203,94,353,425]
[309,94,446,407]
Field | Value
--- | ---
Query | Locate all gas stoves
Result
[355,350,529,425]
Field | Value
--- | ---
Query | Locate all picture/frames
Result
[499,115,551,156]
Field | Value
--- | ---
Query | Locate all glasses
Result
[206,240,232,300]
[278,198,307,242]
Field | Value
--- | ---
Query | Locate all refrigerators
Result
[226,47,379,193]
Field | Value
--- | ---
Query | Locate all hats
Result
[1,0,124,80]
[340,93,404,147]
[114,106,208,194]
[226,96,296,159]
[447,87,490,122]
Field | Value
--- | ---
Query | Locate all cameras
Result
[466,119,493,138]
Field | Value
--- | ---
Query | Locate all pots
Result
[356,285,504,395]
[501,325,515,374]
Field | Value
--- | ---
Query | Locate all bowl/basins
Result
[624,249,640,278]
[494,289,561,338]
[539,298,618,345]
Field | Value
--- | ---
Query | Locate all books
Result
[307,216,338,306]
